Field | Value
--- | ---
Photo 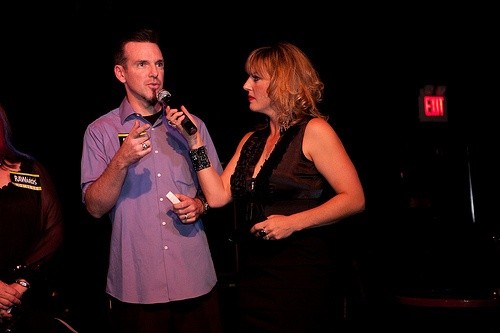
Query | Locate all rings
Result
[259,229,266,236]
[142,142,148,149]
[185,214,188,219]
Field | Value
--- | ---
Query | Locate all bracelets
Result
[189,146,211,172]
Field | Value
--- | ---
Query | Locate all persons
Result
[165,40,365,333]
[0,106,64,333]
[397,80,474,293]
[81,28,224,333]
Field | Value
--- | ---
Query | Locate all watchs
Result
[198,193,210,212]
[15,278,31,288]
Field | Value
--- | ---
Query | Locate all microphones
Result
[155,88,198,136]
